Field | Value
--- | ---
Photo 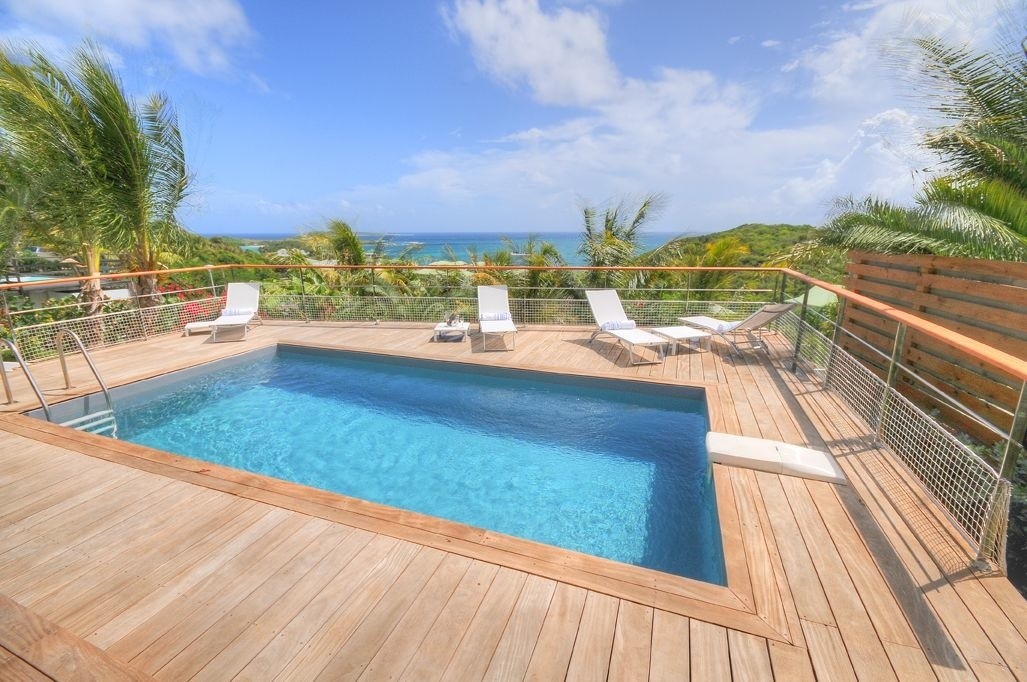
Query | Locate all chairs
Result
[678,304,794,359]
[209,282,264,343]
[477,284,517,352]
[585,289,669,365]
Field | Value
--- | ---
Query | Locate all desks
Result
[184,321,211,336]
[433,322,470,342]
[649,326,712,355]
